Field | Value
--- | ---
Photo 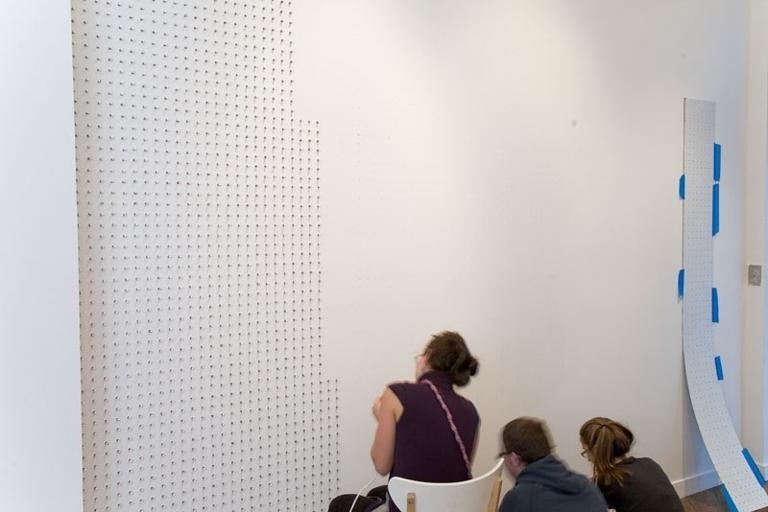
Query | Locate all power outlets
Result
[748,266,762,285]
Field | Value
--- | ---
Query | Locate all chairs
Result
[389,459,504,512]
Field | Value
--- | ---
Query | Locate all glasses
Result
[580,449,589,458]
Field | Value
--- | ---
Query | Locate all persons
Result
[328,331,482,512]
[579,416,685,512]
[498,417,609,512]
